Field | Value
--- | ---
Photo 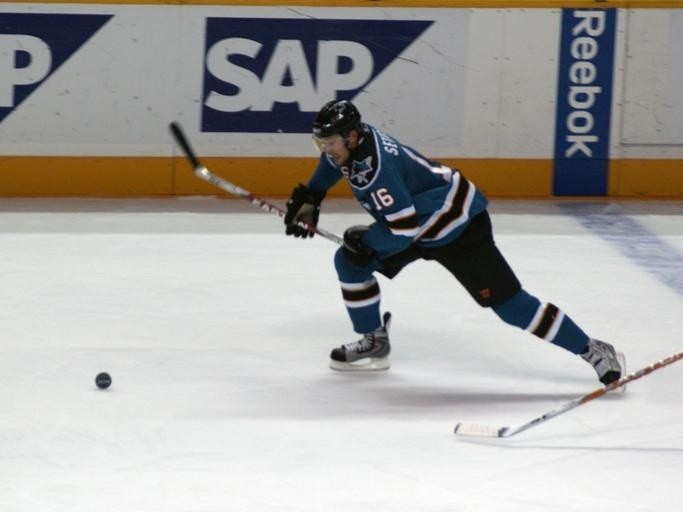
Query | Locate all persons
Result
[283,98,622,384]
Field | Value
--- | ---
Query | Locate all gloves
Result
[284,184,327,238]
[343,225,376,260]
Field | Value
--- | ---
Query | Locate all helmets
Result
[311,99,362,153]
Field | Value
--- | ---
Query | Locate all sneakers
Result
[331,328,390,361]
[581,337,621,385]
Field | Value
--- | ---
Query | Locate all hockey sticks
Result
[454,351,683,439]
[171,124,347,246]
